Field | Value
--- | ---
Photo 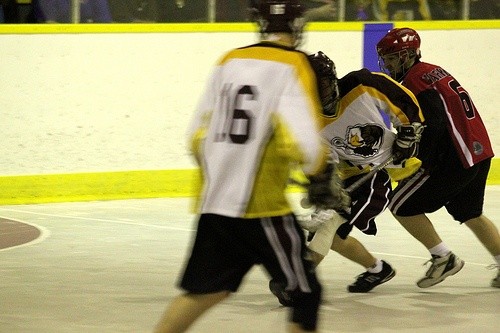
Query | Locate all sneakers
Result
[417,251,464,289]
[490,270,500,287]
[269,280,293,307]
[348,260,396,292]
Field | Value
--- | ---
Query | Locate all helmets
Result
[249,0,304,48]
[376,28,421,82]
[308,51,339,111]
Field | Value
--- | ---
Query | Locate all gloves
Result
[307,164,350,210]
[391,125,424,165]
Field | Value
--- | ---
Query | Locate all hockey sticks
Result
[295,126,425,231]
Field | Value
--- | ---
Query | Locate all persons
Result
[154,1,329,332]
[376,28,500,288]
[372,0,432,22]
[267,50,427,309]
